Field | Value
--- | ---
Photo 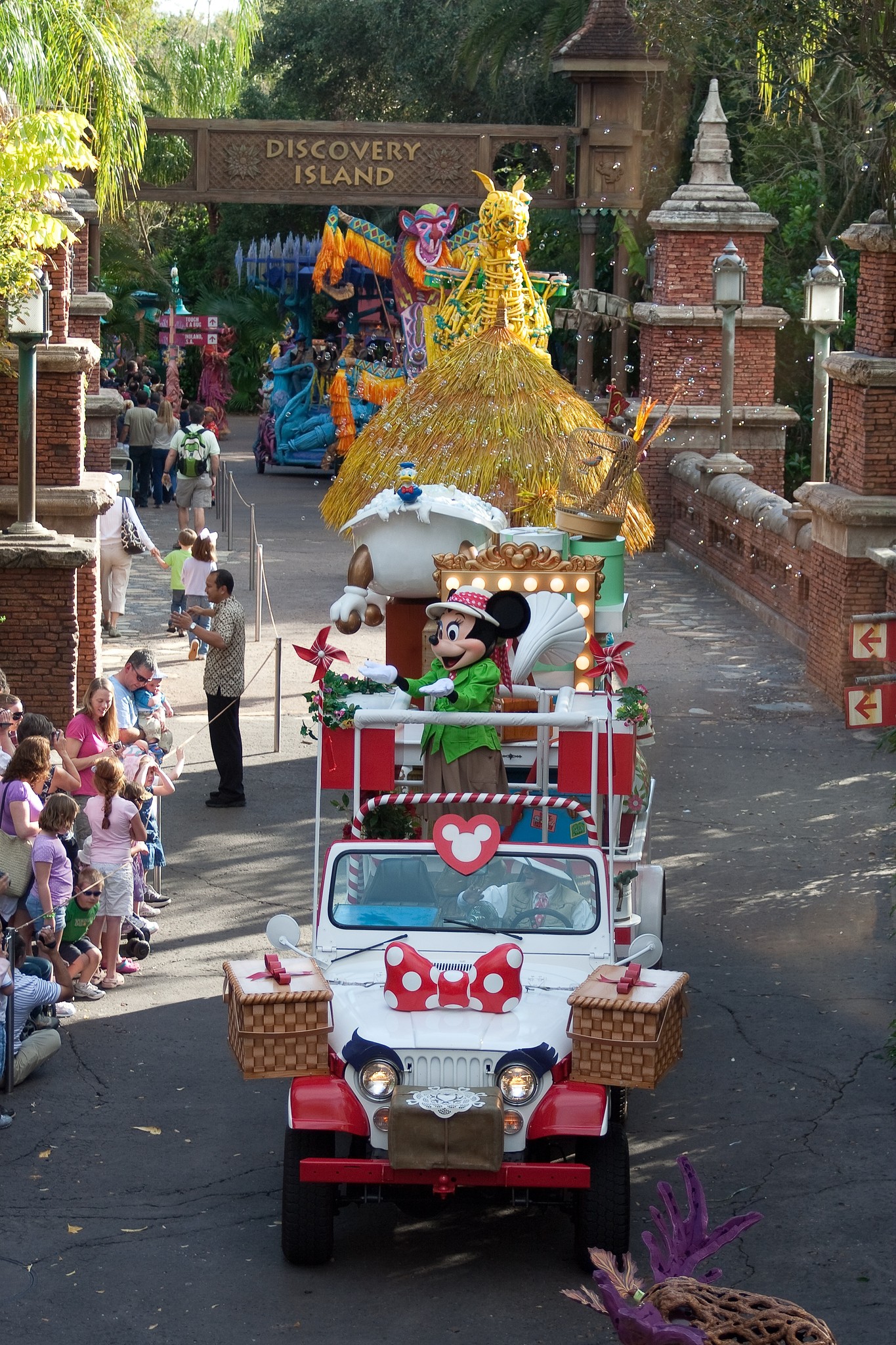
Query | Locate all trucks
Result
[283,685,667,1276]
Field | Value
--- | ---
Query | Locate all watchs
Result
[188,622,196,632]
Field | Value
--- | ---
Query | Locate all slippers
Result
[167,626,187,637]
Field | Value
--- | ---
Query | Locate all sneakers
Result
[29,1013,60,1030]
[48,1001,76,1017]
[120,884,171,936]
[101,618,122,637]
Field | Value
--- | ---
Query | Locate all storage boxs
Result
[557,687,636,795]
[320,683,411,792]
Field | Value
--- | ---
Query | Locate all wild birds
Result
[575,456,603,467]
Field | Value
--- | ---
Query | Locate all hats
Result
[152,669,168,679]
[109,473,123,482]
[204,406,216,415]
[135,788,153,801]
[143,369,153,377]
[103,379,120,388]
[78,834,92,865]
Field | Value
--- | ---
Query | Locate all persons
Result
[290,332,382,404]
[0,359,247,1129]
[457,857,596,931]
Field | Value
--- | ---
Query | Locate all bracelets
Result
[42,912,56,919]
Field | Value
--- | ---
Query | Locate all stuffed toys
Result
[358,585,531,839]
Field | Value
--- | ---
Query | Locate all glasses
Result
[44,716,50,722]
[131,663,151,683]
[77,885,102,897]
[12,708,24,720]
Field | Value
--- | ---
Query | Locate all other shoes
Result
[73,955,140,999]
[205,791,246,808]
[133,489,163,508]
[173,542,182,549]
[1,1106,16,1118]
[149,744,165,764]
[188,638,207,661]
[0,1114,13,1129]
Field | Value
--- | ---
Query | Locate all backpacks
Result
[179,427,213,476]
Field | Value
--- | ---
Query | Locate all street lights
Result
[0,259,57,540]
[694,237,754,474]
[783,245,846,519]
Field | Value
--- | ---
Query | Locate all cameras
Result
[51,730,59,742]
[36,925,51,943]
[114,741,123,750]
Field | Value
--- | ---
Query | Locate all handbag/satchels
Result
[0,828,33,898]
[121,496,147,554]
